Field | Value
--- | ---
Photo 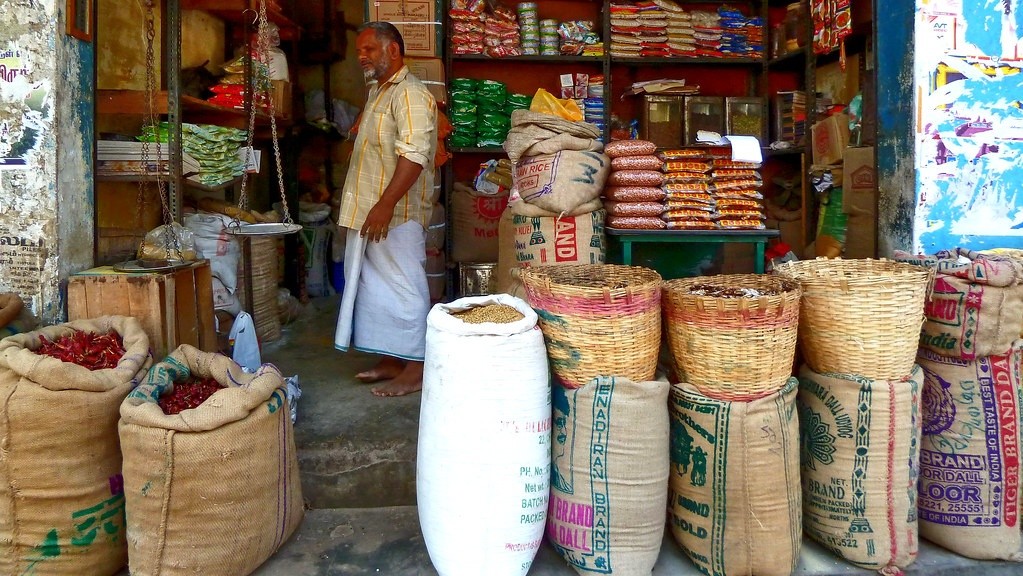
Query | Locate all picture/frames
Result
[66,0,92,42]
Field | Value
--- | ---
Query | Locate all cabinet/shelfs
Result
[96,0,336,353]
[442,0,874,295]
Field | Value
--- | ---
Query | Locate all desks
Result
[605,226,781,274]
[67,259,217,363]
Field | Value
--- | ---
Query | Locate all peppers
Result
[158,376,228,415]
[31,326,125,371]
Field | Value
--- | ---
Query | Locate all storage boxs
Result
[402,57,446,108]
[812,113,852,164]
[843,146,875,215]
[364,0,443,58]
[271,79,293,119]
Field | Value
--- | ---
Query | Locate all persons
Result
[334,20,431,398]
